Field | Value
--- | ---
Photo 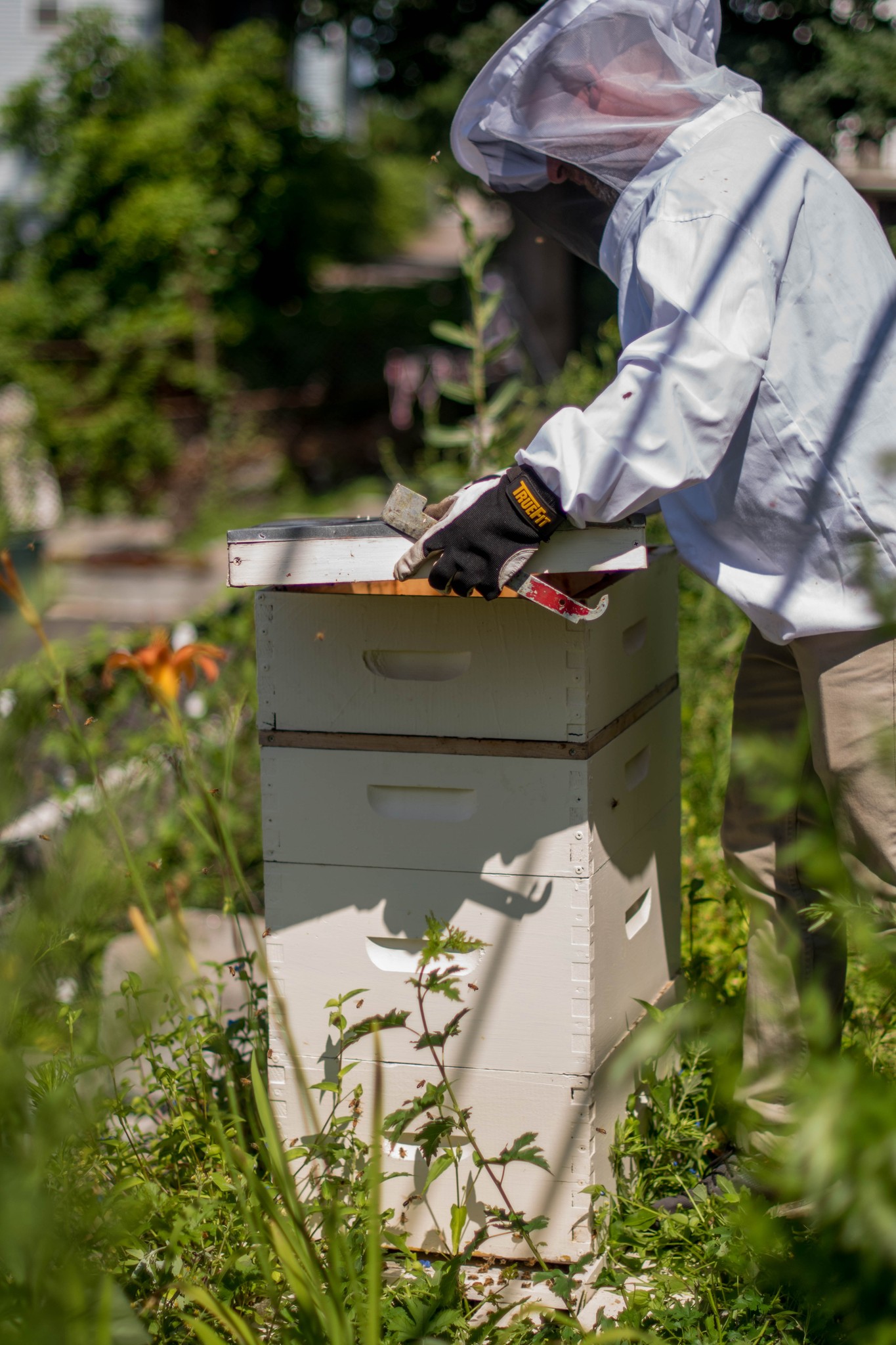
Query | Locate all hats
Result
[450,0,722,193]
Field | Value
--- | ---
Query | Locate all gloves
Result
[393,461,567,601]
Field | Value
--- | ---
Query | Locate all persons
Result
[394,0,896,1253]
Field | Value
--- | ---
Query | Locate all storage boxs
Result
[226,501,684,1265]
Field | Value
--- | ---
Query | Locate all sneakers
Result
[648,1152,852,1227]
